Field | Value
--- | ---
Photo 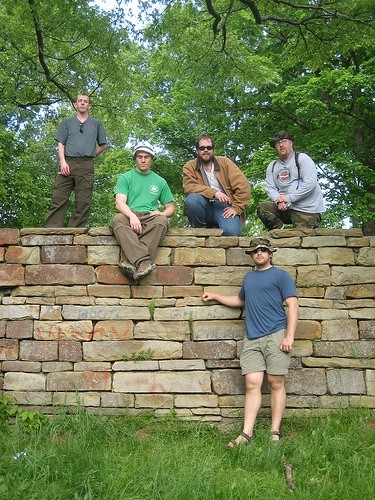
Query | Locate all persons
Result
[202,238,299,449]
[182,135,251,237]
[44,93,107,228]
[257,131,326,230]
[109,141,176,286]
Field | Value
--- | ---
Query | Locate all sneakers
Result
[118,258,140,286]
[133,258,156,280]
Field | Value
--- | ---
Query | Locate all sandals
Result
[225,431,256,449]
[269,431,280,444]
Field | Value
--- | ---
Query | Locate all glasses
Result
[197,146,214,151]
[274,139,291,146]
[79,123,83,134]
[136,155,150,159]
[252,247,268,253]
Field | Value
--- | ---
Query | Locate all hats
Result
[269,131,294,148]
[133,141,157,161]
[245,237,277,255]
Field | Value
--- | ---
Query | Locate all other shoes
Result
[278,223,285,229]
